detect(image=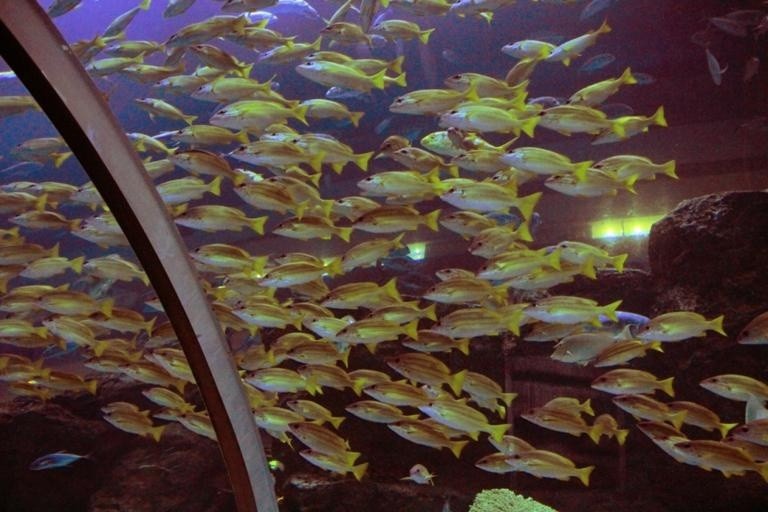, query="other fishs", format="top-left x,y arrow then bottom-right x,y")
0,0 -> 768,488
